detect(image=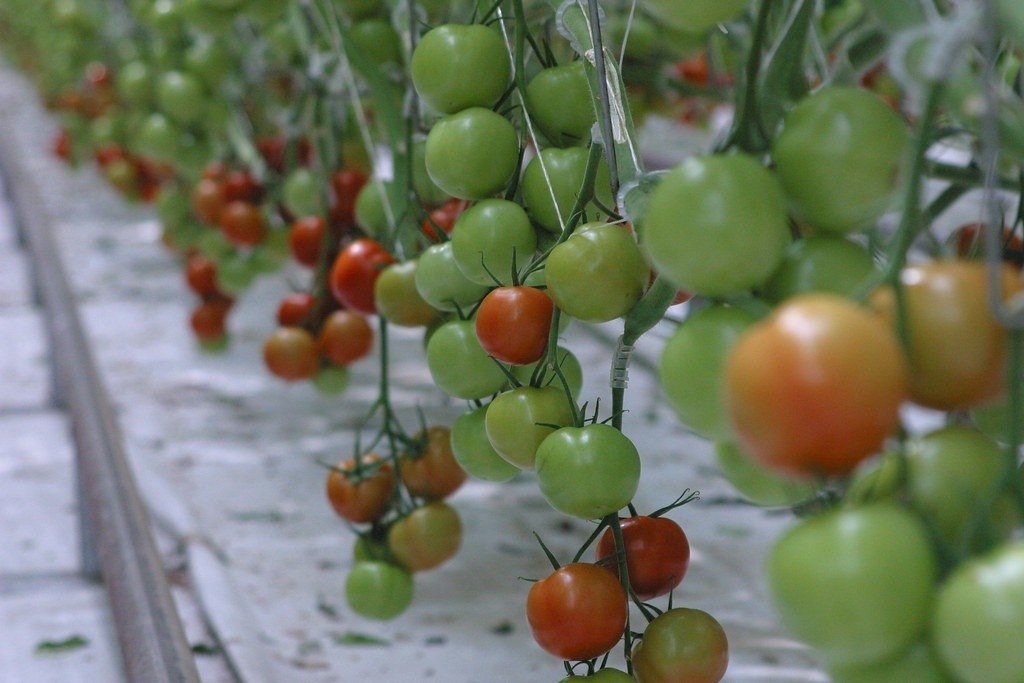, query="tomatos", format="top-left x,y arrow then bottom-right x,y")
0,0 -> 1024,683
